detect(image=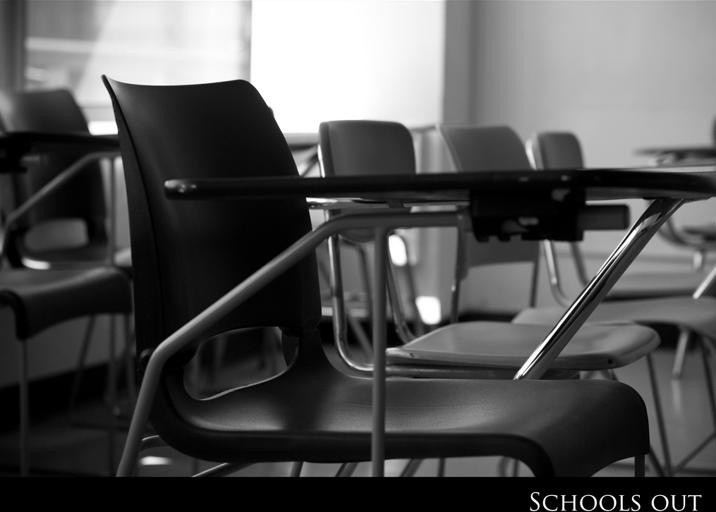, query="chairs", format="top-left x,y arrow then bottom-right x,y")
0,72 -> 715,483
